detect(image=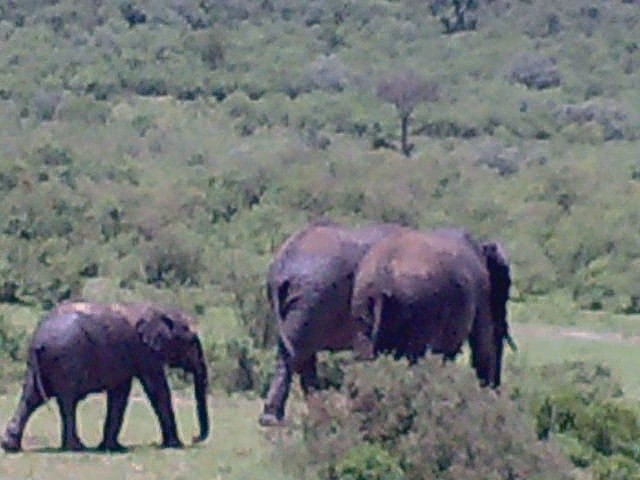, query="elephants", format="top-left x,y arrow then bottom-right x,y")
255,217 -> 421,428
0,299 -> 211,453
349,223 -> 520,397
